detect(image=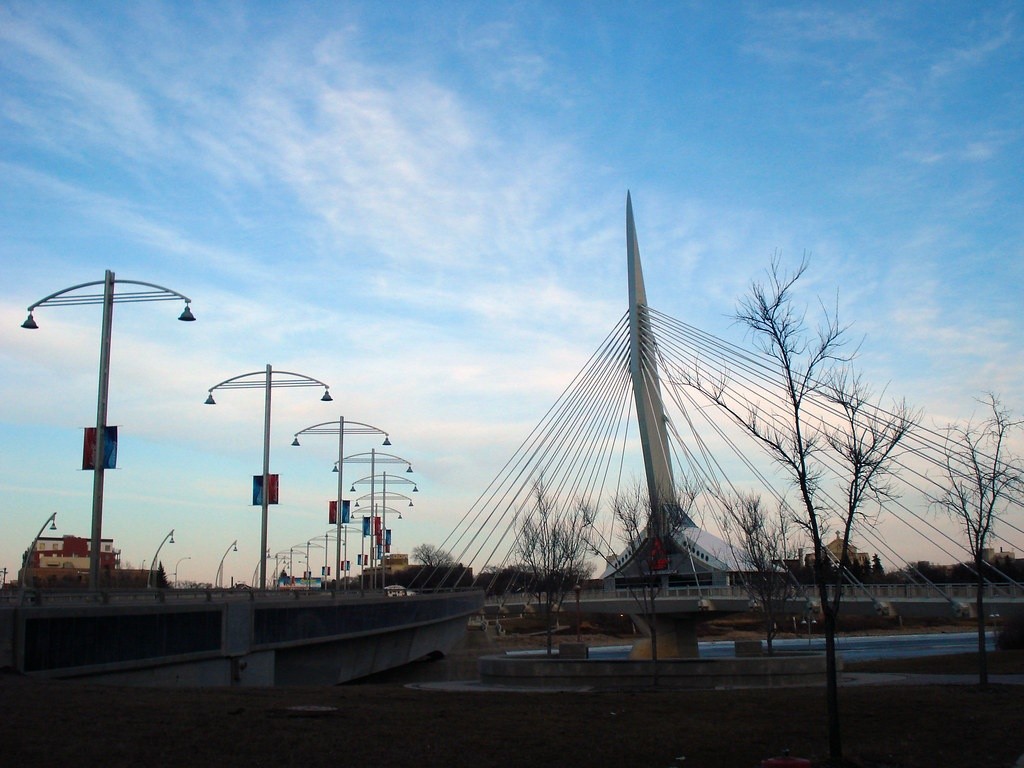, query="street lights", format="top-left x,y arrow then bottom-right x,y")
332,449 -> 419,590
23,271 -> 197,590
144,529 -> 176,590
20,511 -> 57,593
252,547 -> 271,590
214,541 -> 238,587
204,367 -> 333,588
294,420 -> 393,591
